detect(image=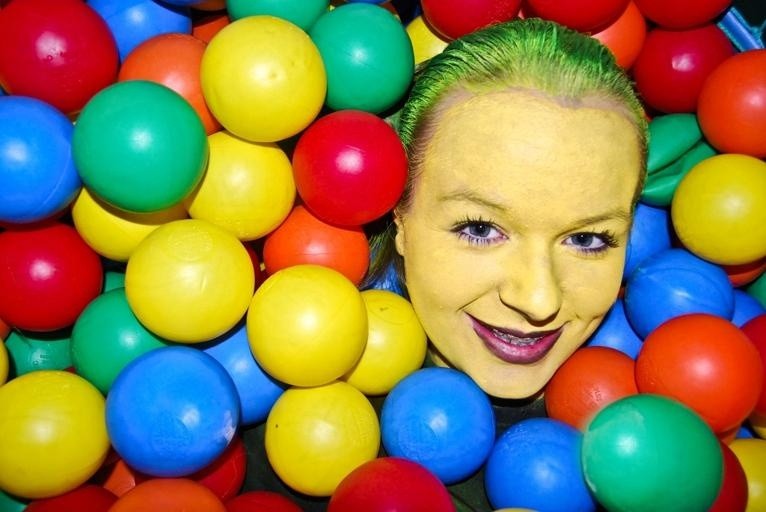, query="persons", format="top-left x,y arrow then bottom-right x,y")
240,16 -> 648,511
